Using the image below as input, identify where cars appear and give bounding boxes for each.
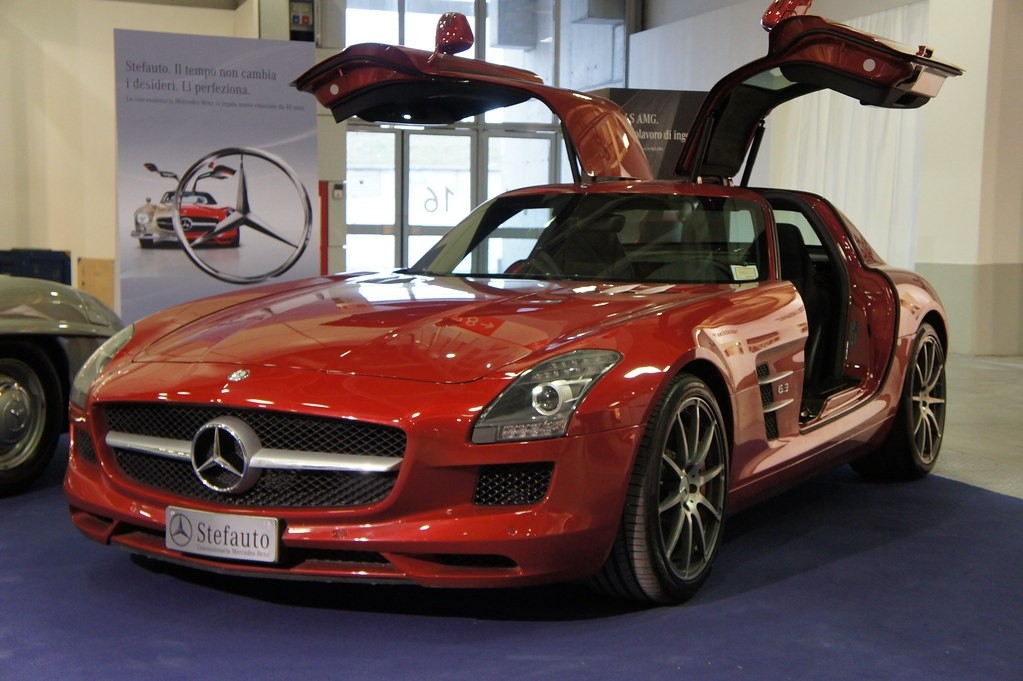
[0,272,130,499]
[129,162,240,249]
[72,0,949,614]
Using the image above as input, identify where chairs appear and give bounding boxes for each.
[535,200,635,280]
[738,222,820,360]
